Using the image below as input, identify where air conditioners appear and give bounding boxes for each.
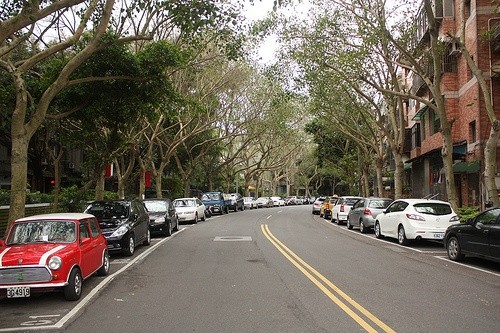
[449,42,459,56]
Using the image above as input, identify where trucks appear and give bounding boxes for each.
[203,192,232,217]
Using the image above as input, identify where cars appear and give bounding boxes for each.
[80,199,150,257]
[243,196,259,210]
[227,193,244,212]
[283,195,316,206]
[144,198,179,238]
[172,198,208,225]
[373,199,459,247]
[320,194,338,220]
[443,207,500,264]
[312,195,325,214]
[271,197,286,207]
[346,198,395,233]
[256,197,274,208]
[330,196,363,225]
[0,213,110,303]
